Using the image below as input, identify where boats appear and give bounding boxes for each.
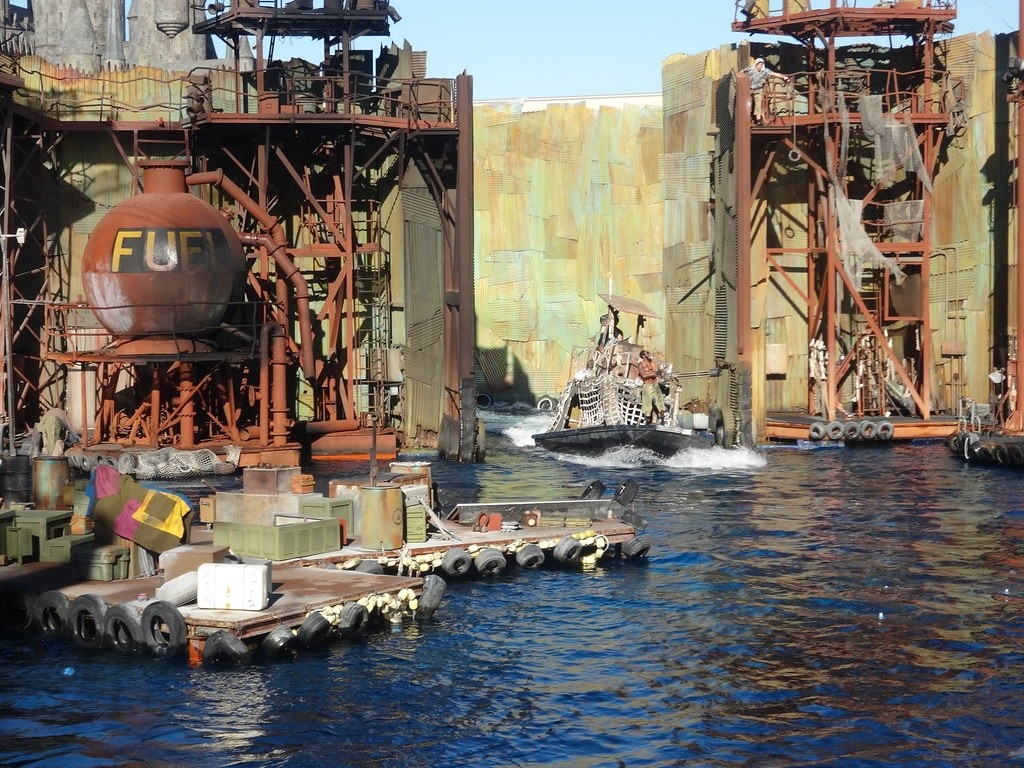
[533,298,720,467]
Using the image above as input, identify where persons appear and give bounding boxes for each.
[639,350,667,425]
[740,57,790,126]
